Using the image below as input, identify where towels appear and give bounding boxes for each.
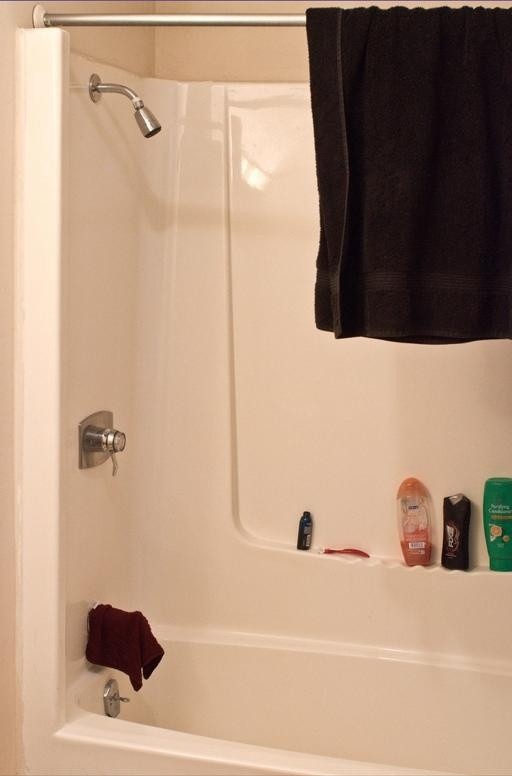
[86,603,164,692]
[301,8,511,342]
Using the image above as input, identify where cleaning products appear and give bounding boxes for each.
[297,512,312,549]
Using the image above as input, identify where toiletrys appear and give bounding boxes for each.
[296,510,313,551]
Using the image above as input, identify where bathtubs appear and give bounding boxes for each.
[56,623,508,773]
